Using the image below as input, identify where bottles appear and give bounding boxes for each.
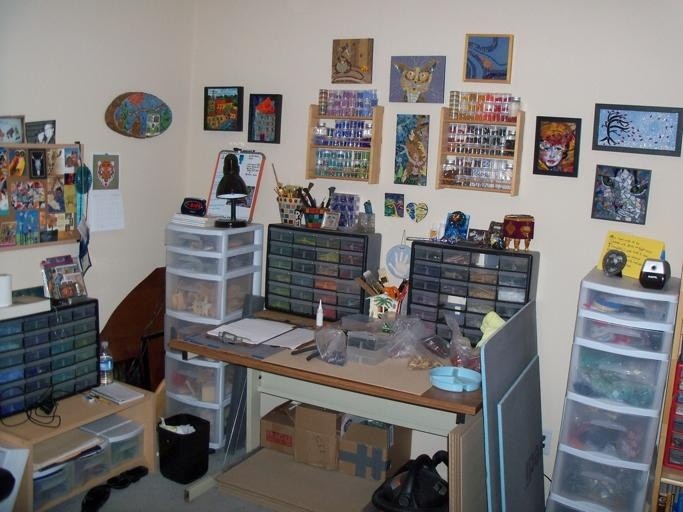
[447,124,514,155]
[314,120,372,148]
[100,340,113,384]
[443,156,513,190]
[314,149,369,179]
[449,90,520,123]
[318,88,377,117]
[316,299,323,327]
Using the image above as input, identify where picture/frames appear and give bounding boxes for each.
[591,103,683,157]
[0,143,86,250]
[462,32,515,85]
[532,115,581,178]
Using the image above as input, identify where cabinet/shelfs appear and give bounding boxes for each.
[434,106,525,197]
[0,380,157,512]
[540,267,683,511]
[162,211,264,457]
[305,104,384,186]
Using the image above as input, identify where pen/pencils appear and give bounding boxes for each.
[291,347,317,355]
[276,186,301,197]
[306,353,318,361]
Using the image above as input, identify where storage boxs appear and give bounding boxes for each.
[258,396,415,483]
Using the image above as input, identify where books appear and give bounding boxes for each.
[91,381,146,408]
[39,253,89,301]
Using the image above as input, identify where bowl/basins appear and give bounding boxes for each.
[428,366,481,392]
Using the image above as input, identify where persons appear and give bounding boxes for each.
[0,122,81,186]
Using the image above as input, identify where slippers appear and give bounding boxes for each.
[83,486,110,511]
[105,466,148,490]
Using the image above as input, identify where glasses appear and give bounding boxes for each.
[218,331,256,350]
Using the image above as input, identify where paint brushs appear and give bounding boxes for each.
[303,183,314,200]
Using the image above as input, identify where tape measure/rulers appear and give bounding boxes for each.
[355,277,376,296]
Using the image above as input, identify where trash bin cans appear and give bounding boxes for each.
[157,413,209,484]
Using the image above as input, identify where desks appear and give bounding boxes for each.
[168,310,484,512]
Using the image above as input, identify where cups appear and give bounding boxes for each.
[0,274,12,307]
